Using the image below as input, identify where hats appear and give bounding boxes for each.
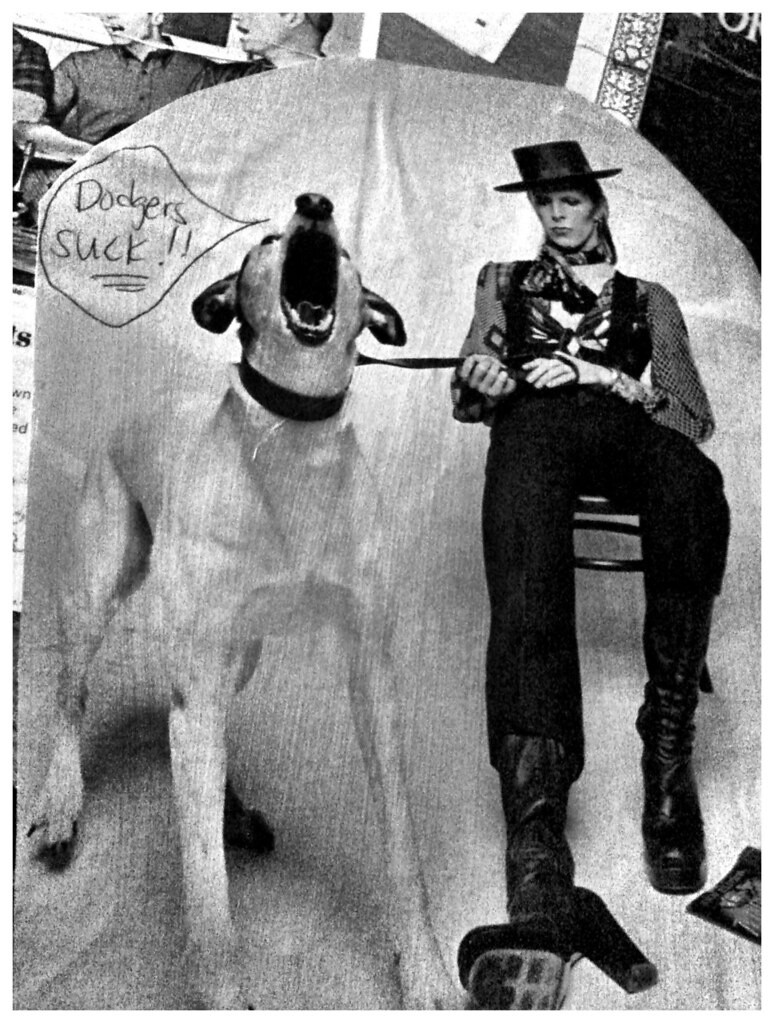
[493,139,622,193]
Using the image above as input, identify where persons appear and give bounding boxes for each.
[447,140,731,921]
[12,13,337,227]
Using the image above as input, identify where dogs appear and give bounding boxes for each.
[26,191,474,1010]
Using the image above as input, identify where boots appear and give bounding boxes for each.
[635,594,711,892]
[457,731,657,1009]
